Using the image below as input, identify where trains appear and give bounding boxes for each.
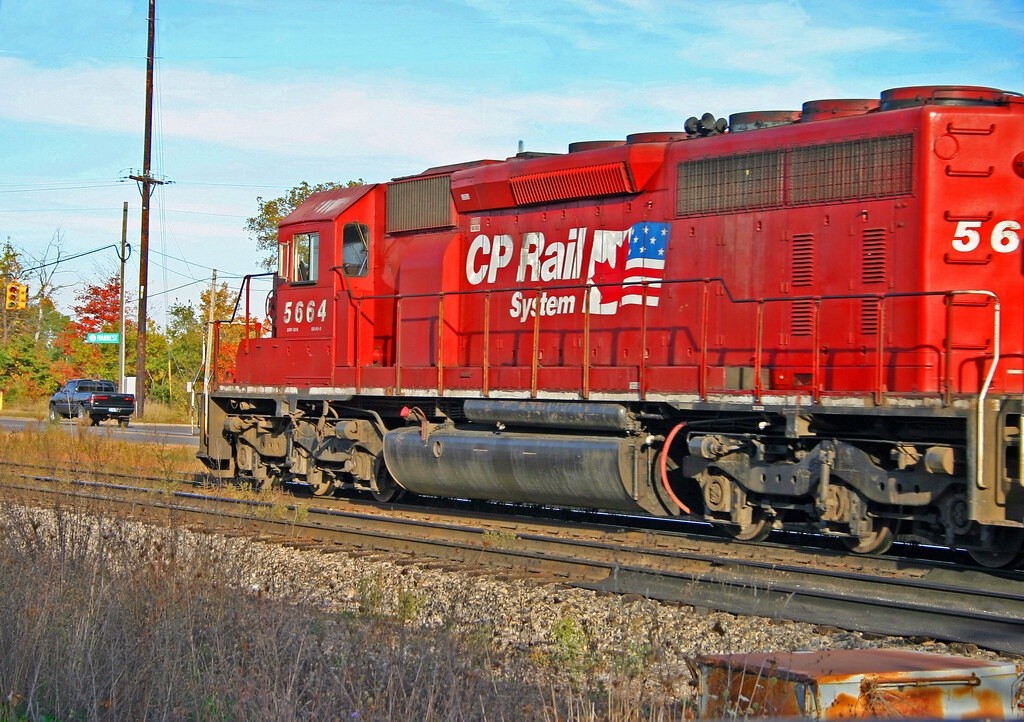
[199,88,1024,570]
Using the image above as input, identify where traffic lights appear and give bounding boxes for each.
[18,285,29,310]
[6,283,18,310]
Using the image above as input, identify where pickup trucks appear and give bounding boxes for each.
[49,382,135,430]
